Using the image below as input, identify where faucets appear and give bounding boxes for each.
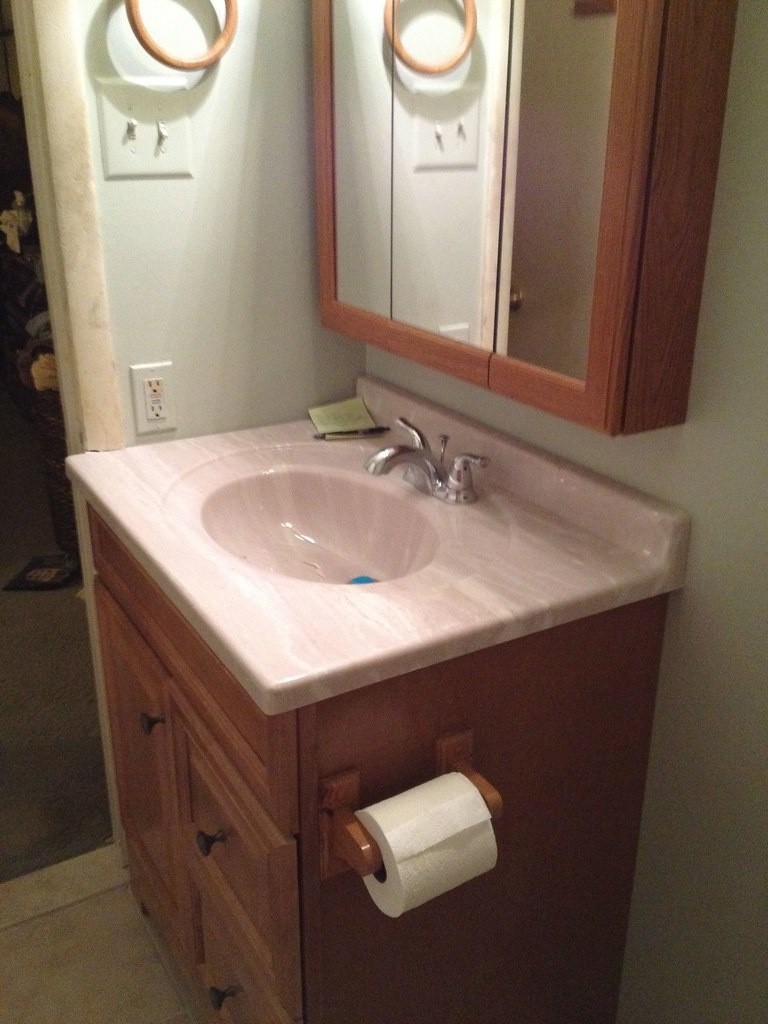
[361,416,492,505]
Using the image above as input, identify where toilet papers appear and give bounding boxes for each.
[353,771,499,919]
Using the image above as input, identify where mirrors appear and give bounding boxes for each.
[300,0,738,441]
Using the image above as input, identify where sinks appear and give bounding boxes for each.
[199,470,443,586]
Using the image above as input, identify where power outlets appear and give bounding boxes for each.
[129,356,180,440]
[438,321,472,347]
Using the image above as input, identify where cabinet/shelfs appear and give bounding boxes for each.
[80,499,676,1024]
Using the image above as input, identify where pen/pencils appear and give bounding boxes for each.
[314,426,390,439]
